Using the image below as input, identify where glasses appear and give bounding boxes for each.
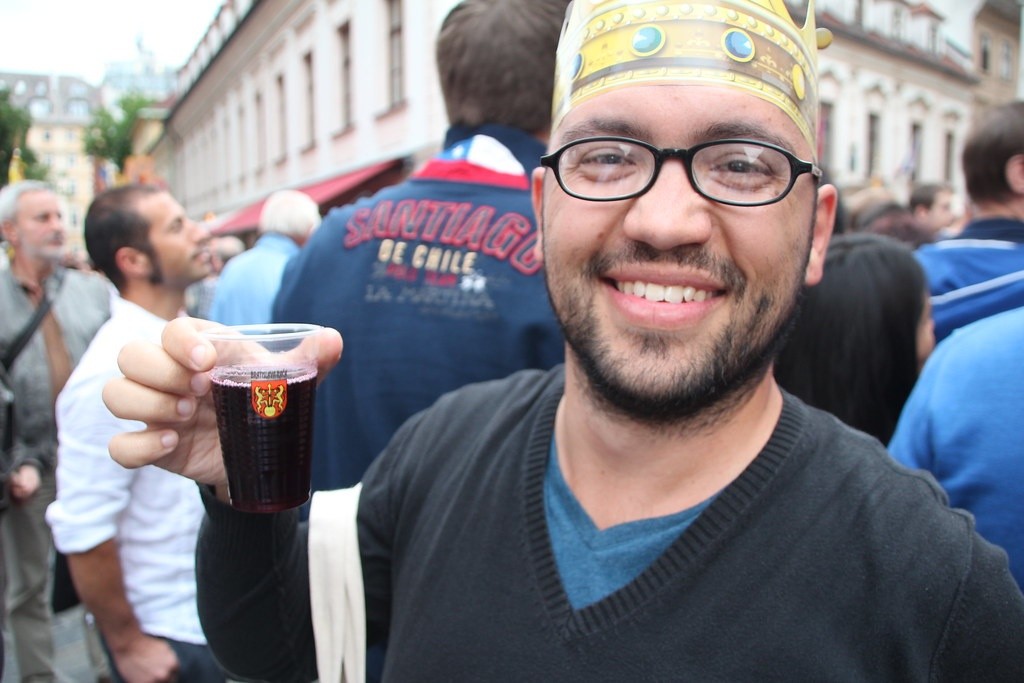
[542,137,824,208]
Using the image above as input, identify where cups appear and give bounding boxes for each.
[198,323,325,513]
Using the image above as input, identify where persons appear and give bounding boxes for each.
[0,0,1024,683]
